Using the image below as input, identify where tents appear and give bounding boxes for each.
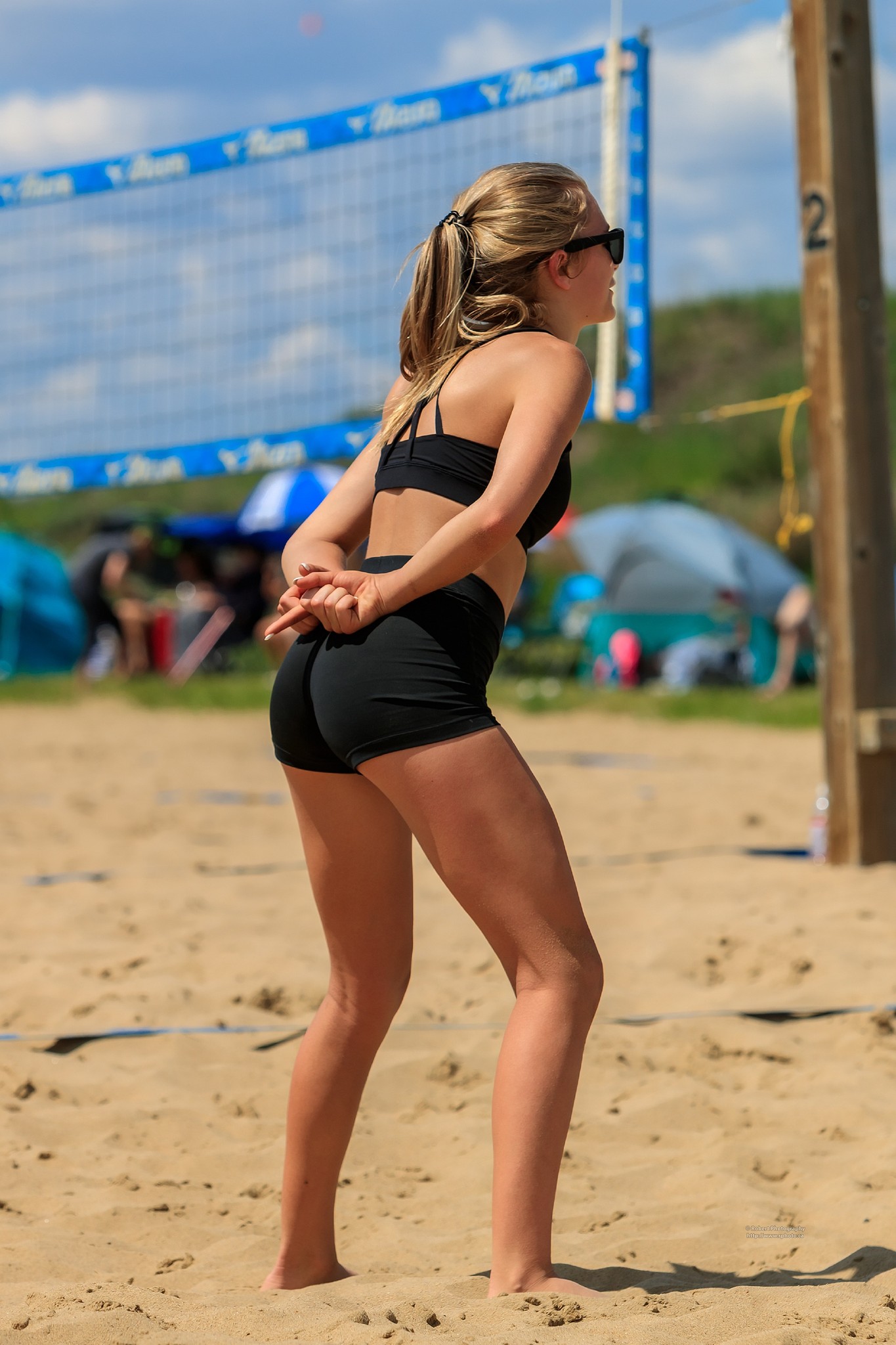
[562,501,820,685]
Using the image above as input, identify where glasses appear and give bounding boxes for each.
[520,226,625,277]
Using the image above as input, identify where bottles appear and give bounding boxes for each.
[811,786,829,860]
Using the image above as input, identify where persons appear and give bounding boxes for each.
[265,162,624,1298]
[72,526,301,685]
